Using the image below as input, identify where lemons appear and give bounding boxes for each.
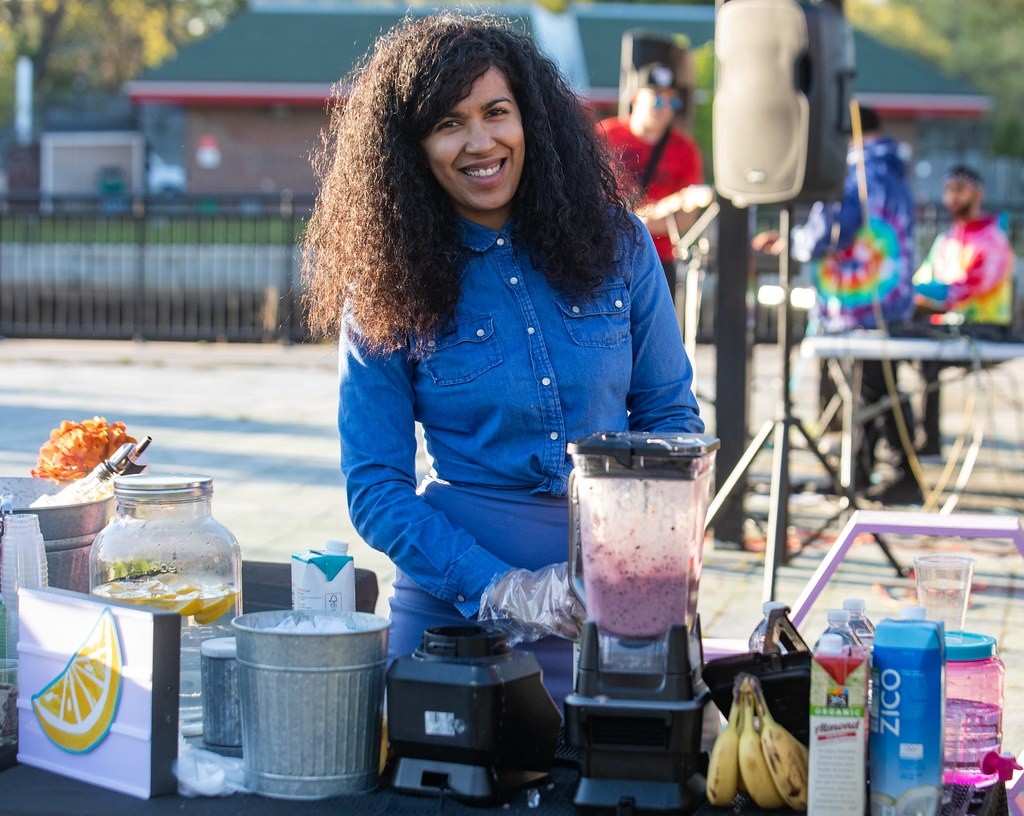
[107,579,239,624]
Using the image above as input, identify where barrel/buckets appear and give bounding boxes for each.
[234,612,391,799]
[0,476,114,591]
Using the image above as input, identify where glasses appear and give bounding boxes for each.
[650,94,683,112]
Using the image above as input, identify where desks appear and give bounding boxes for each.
[0,611,784,816]
[809,335,1024,518]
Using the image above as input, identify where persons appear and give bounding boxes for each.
[814,103,927,506]
[752,198,850,433]
[904,163,1016,457]
[585,59,702,310]
[305,8,709,717]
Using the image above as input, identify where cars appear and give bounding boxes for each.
[676,218,807,347]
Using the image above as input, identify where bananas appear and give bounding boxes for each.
[705,672,809,811]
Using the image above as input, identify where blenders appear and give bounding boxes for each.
[563,430,719,809]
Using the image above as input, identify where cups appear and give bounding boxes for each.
[913,554,974,632]
[0,514,49,772]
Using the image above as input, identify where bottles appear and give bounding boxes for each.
[808,611,869,816]
[870,609,1006,816]
[841,597,874,784]
[748,603,786,654]
[88,475,241,737]
